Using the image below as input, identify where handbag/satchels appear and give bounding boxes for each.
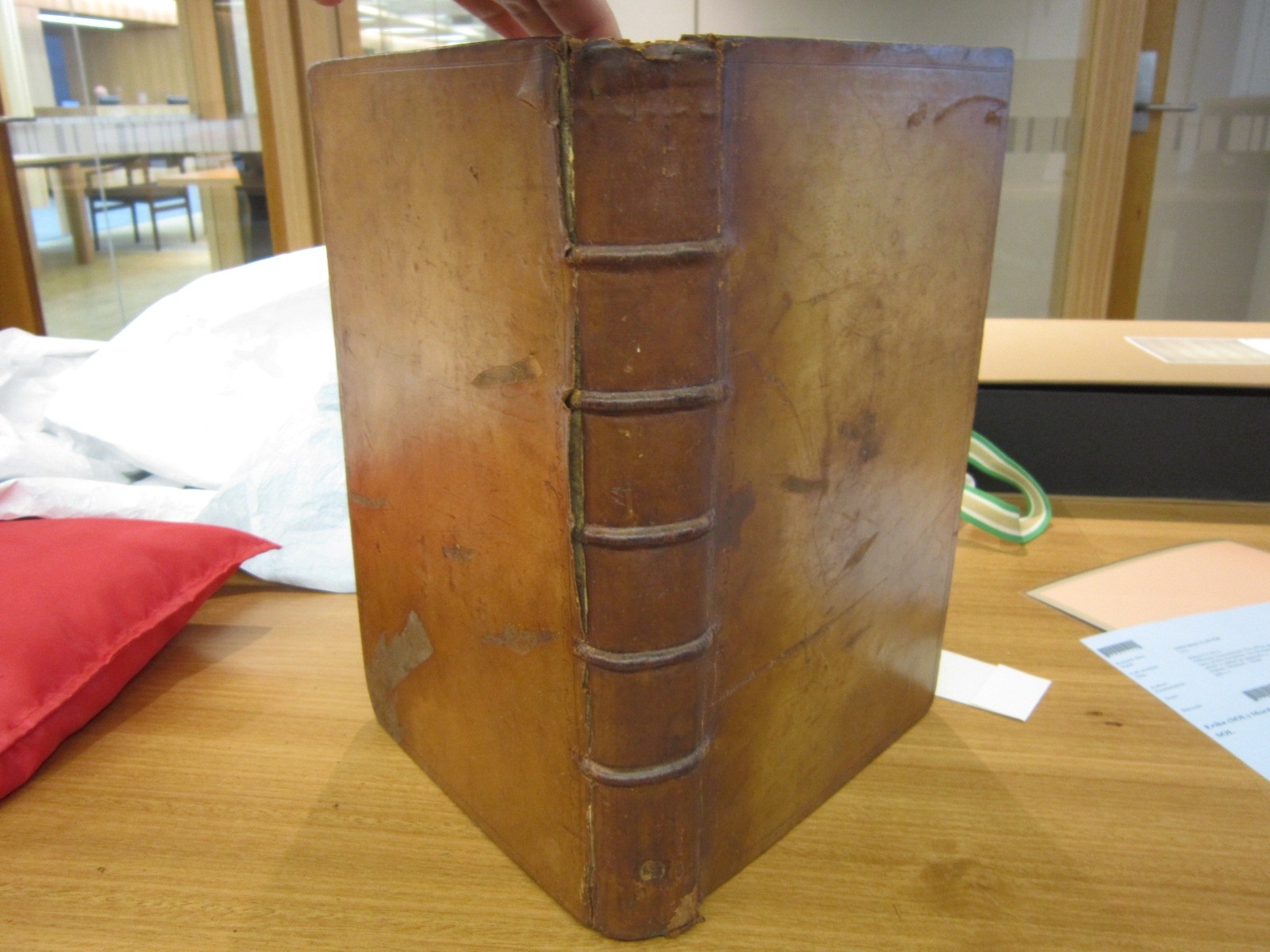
[36,242,1052,544]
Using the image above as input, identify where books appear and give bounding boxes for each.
[305,33,1015,941]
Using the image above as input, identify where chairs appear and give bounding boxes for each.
[86,155,196,249]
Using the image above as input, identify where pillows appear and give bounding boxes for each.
[1,518,281,800]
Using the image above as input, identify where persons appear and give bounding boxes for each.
[91,84,110,104]
